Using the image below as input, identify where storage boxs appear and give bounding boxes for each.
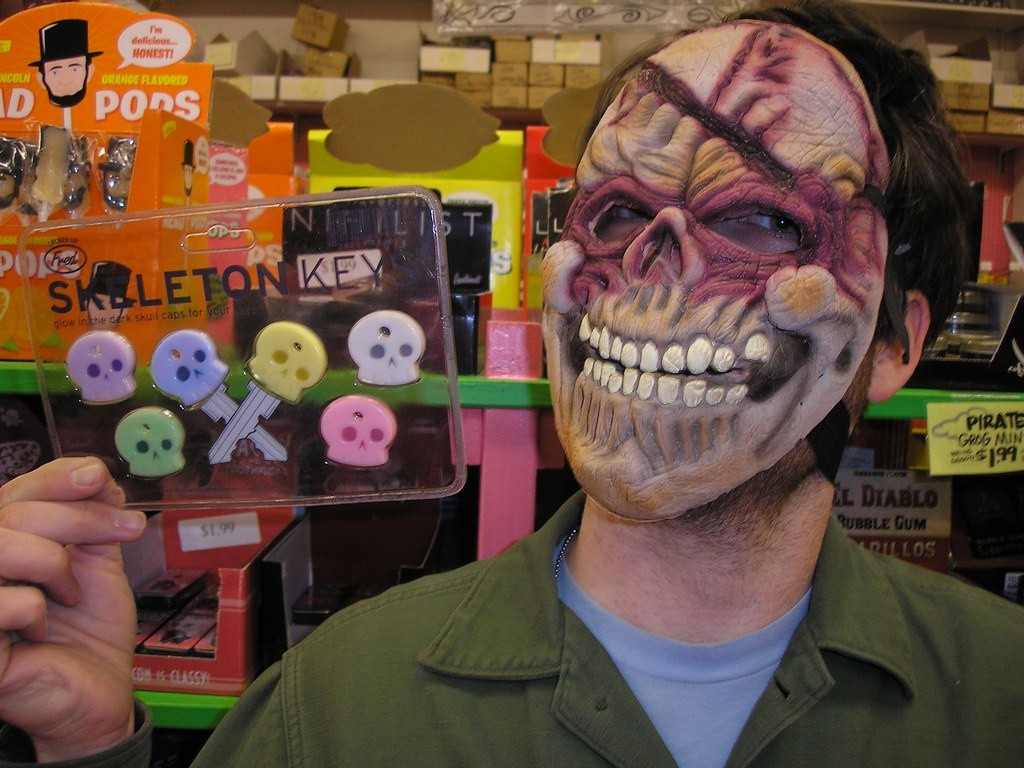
[200,3,618,375]
[120,502,313,699]
[909,26,1024,140]
[0,0,214,365]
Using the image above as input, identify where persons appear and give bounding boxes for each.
[1,0,1024,767]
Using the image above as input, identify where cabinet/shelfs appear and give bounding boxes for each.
[0,360,1024,733]
